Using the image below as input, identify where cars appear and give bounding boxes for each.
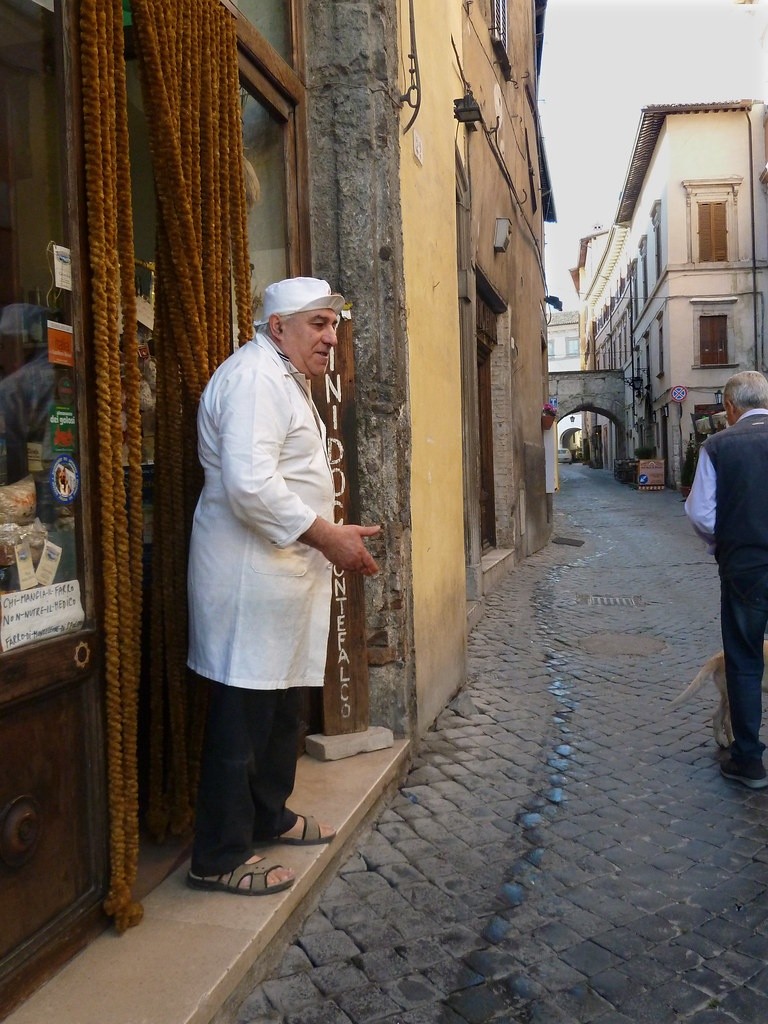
[558,448,572,464]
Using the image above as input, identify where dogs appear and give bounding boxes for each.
[666,638,768,747]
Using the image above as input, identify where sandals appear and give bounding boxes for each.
[257,813,337,844]
[186,854,294,893]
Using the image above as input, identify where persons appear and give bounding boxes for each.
[684,370,768,789]
[186,276,382,896]
[0,303,76,580]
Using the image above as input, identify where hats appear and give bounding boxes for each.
[254,277,345,327]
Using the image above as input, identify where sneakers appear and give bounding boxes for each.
[719,756,768,788]
